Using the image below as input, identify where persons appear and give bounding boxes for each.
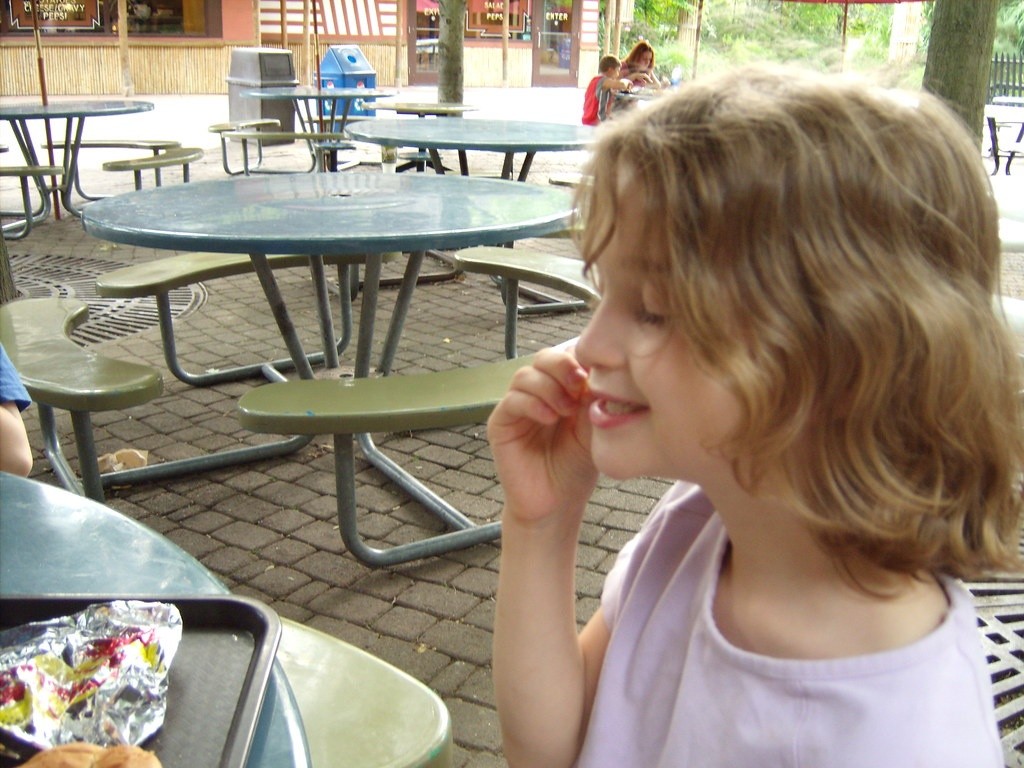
[484,66,1024,767]
[0,342,33,478]
[606,40,662,115]
[581,55,633,127]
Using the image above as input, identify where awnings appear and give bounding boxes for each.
[416,0,440,15]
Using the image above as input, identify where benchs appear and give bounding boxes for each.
[1,104,603,568]
[275,617,452,768]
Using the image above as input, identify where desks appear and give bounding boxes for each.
[0,101,154,198]
[252,88,394,169]
[345,118,601,274]
[0,469,311,768]
[80,171,585,376]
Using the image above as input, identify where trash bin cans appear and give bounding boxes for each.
[315,44,378,139]
[224,47,300,146]
